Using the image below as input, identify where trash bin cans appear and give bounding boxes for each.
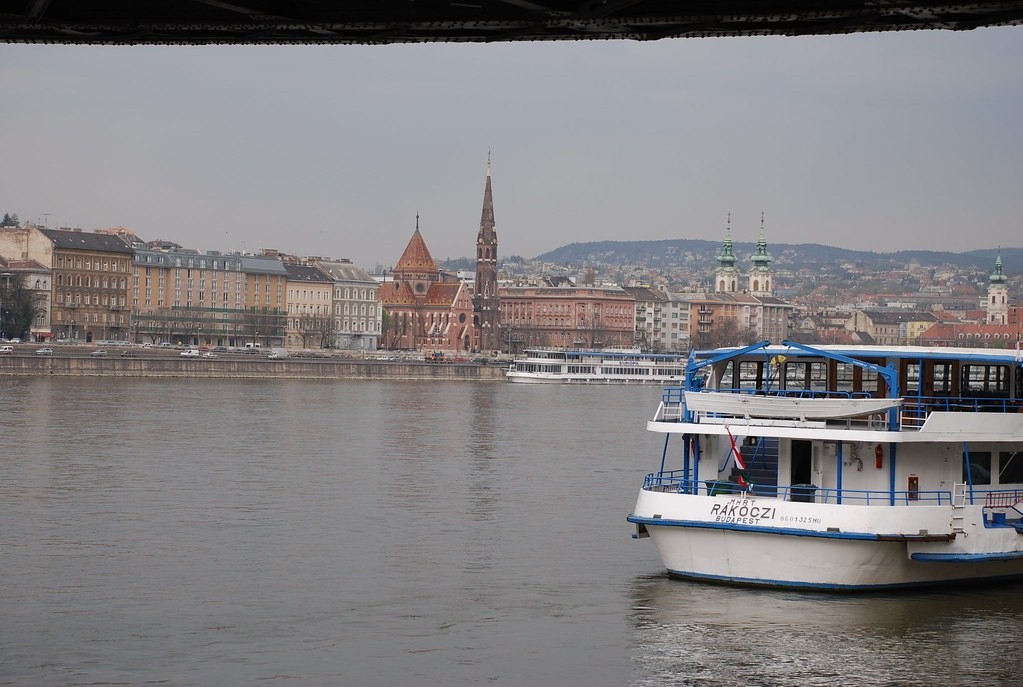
[790,484,818,503]
[705,480,733,496]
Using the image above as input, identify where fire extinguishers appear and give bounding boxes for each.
[875,443,882,468]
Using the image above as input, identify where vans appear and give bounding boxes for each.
[179,349,200,358]
[246,342,261,349]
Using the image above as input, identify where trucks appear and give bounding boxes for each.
[269,347,288,360]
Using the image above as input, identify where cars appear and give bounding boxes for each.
[162,342,171,348]
[119,341,132,347]
[105,340,116,346]
[364,355,425,361]
[198,347,209,352]
[202,352,218,360]
[144,343,153,346]
[176,344,191,350]
[90,349,107,357]
[211,346,226,353]
[0,337,22,344]
[36,348,53,356]
[122,350,137,358]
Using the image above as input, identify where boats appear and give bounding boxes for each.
[722,363,817,388]
[505,348,711,386]
[623,339,1023,596]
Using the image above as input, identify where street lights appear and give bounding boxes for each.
[226,324,230,355]
[198,328,201,347]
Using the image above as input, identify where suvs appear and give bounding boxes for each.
[0,346,14,355]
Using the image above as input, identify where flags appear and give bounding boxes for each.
[729,432,754,491]
